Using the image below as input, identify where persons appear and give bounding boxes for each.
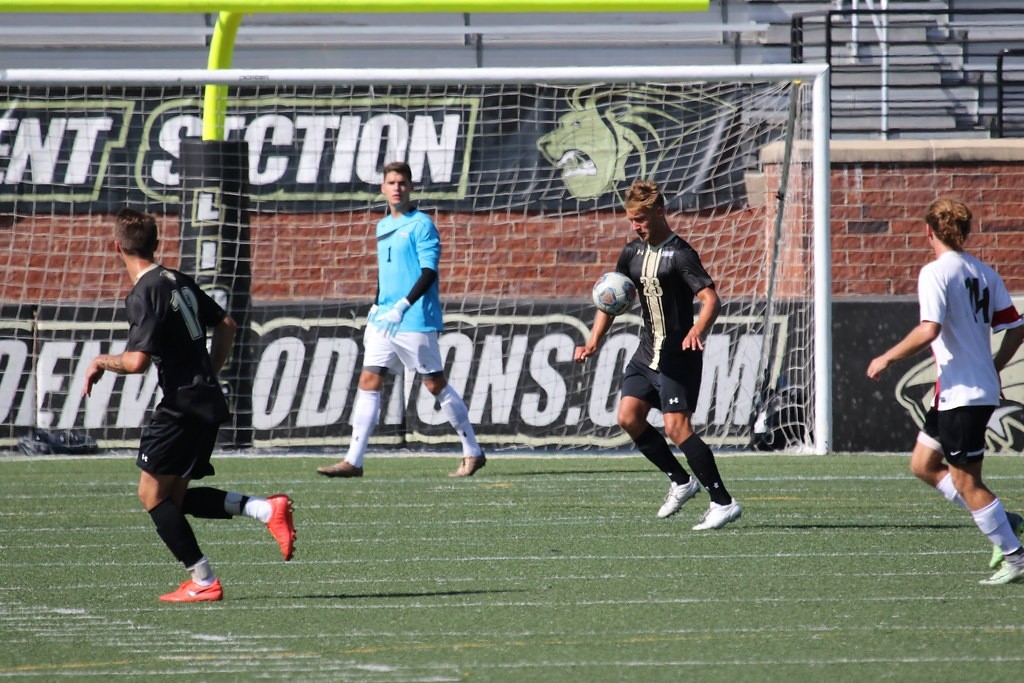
[574,182,741,531]
[317,163,486,479]
[868,197,1023,587]
[83,211,297,602]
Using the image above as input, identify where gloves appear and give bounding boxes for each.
[367,303,379,321]
[376,296,412,338]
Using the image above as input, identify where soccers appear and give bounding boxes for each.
[592,271,636,316]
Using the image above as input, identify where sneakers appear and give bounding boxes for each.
[160,577,223,602]
[691,496,742,530]
[656,475,701,519]
[977,511,1024,586]
[447,449,487,477]
[317,460,364,478]
[265,494,298,562]
